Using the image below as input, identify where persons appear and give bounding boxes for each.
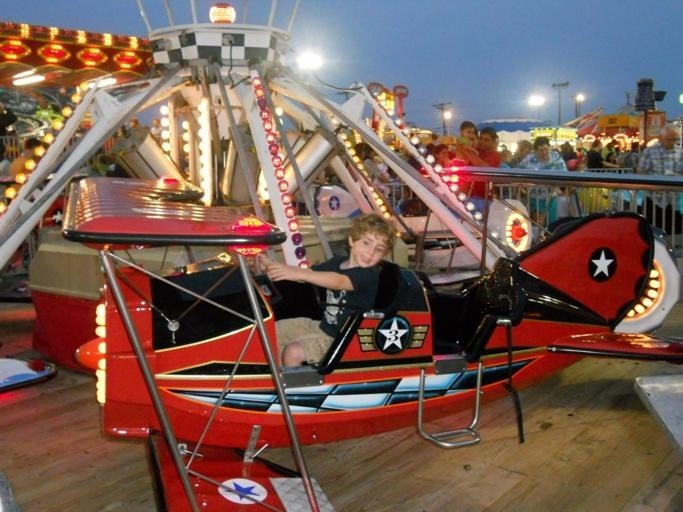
[8,136,40,202]
[265,212,399,371]
[0,142,12,197]
